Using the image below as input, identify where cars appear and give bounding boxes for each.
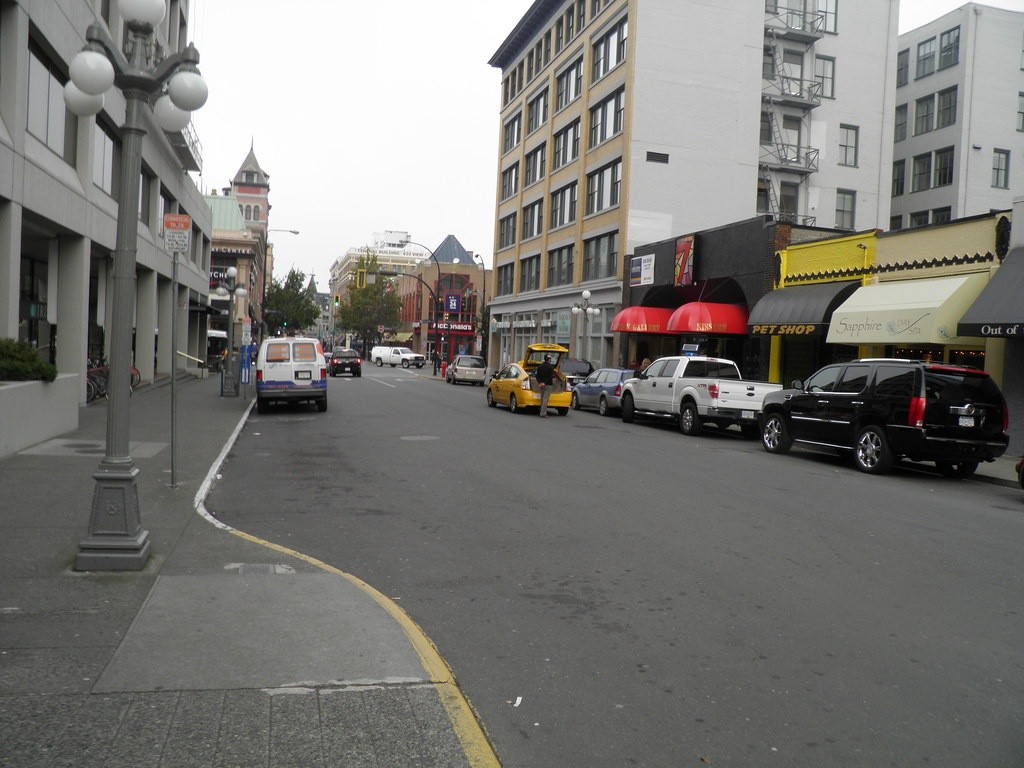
[486,343,572,416]
[539,358,595,392]
[445,356,486,387]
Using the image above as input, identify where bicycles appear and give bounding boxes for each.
[86,356,140,403]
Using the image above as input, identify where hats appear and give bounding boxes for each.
[544,354,552,358]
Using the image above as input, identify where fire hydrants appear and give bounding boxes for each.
[440,362,447,378]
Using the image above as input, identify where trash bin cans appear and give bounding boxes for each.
[225,352,242,395]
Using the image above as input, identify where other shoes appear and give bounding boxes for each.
[540,415,549,418]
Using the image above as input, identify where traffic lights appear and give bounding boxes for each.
[334,295,340,306]
[282,320,287,326]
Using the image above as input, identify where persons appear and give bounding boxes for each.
[430,347,441,375]
[639,357,651,370]
[535,354,554,418]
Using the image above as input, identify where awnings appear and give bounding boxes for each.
[609,246,1024,359]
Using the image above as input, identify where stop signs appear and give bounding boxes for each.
[378,326,384,332]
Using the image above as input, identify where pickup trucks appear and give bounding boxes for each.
[371,346,426,369]
[328,349,362,377]
[620,356,783,440]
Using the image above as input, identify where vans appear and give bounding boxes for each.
[205,330,227,372]
[257,338,328,414]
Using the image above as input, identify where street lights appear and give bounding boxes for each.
[259,229,300,344]
[475,254,485,358]
[400,240,441,377]
[571,289,600,359]
[61,1,210,570]
[216,267,248,397]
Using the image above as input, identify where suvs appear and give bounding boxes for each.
[572,366,638,416]
[756,359,1010,479]
